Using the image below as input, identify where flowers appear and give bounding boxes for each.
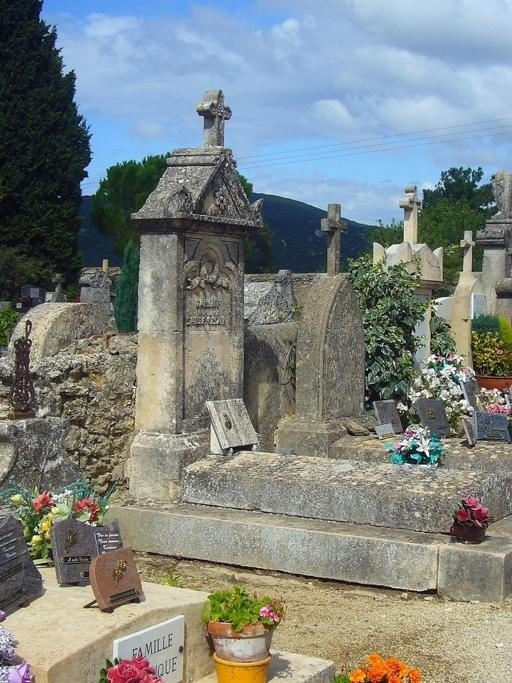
[202,584,281,633]
[337,653,420,682]
[383,424,446,465]
[0,609,35,683]
[453,495,490,530]
[10,483,119,568]
[99,655,163,682]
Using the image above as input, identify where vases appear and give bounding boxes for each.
[212,653,275,683]
[204,621,273,664]
[452,528,484,543]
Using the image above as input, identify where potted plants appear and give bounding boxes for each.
[472,330,512,391]
